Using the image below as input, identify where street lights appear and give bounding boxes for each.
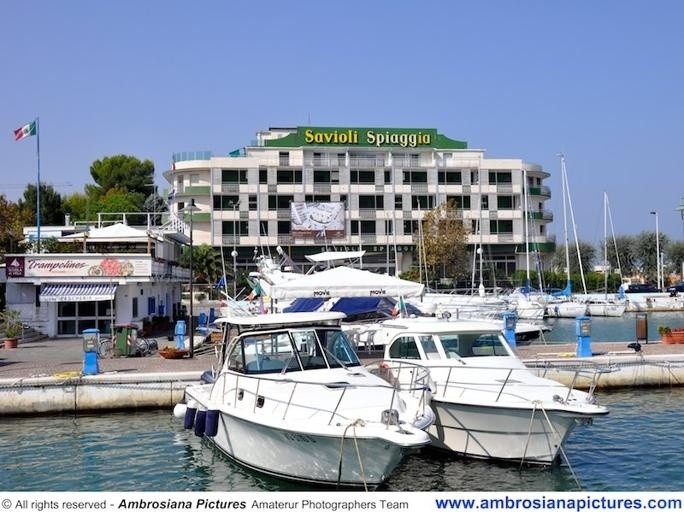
[182,198,204,353]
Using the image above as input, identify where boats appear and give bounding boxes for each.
[171,311,442,493]
[354,308,616,475]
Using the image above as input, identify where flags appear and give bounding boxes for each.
[13,120,36,143]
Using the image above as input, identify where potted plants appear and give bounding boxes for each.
[671,326,683,344]
[656,325,674,344]
[1,305,24,350]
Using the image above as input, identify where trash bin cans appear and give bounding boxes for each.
[635,313,649,344]
[114,322,139,358]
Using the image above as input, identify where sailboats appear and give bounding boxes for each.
[221,154,684,343]
